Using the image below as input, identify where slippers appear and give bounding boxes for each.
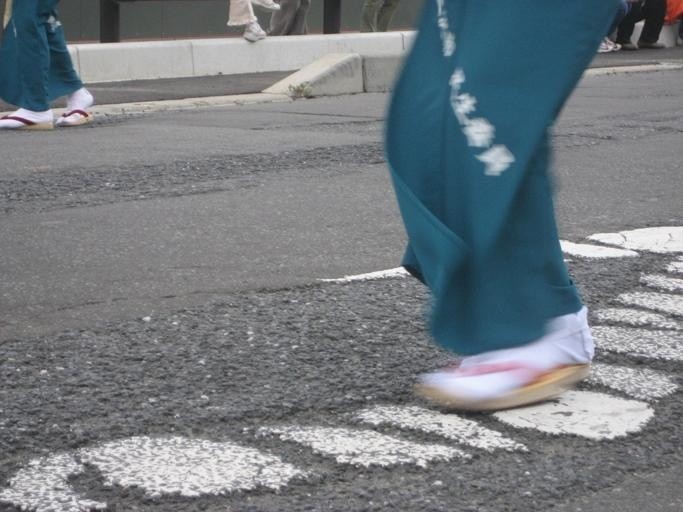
[415,363,591,411]
[1,110,92,129]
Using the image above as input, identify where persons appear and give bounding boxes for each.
[227,0,281,41]
[383,0,632,412]
[595,0,682,54]
[0,0,94,131]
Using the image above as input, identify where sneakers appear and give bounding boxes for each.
[676,34,682,44]
[243,1,281,41]
[597,37,665,53]
